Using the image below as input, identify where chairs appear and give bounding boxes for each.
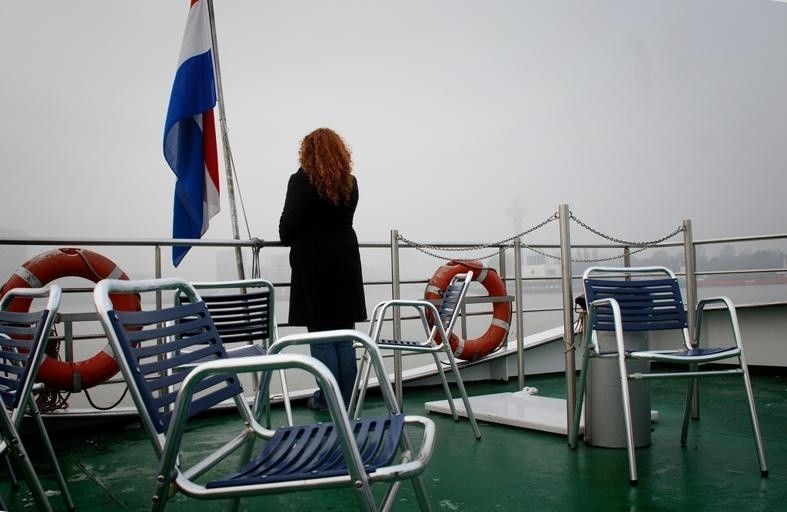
[0,281,76,512]
[164,277,296,434]
[346,267,484,444]
[91,274,439,511]
[566,264,772,488]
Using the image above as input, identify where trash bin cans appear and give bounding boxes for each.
[576,295,651,448]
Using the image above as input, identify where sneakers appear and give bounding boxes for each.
[307,395,350,410]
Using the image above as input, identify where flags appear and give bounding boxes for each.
[163,0,221,268]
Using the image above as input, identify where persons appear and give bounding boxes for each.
[280,128,367,412]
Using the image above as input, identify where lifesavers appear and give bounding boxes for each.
[425,259,510,361]
[0,248,143,392]
